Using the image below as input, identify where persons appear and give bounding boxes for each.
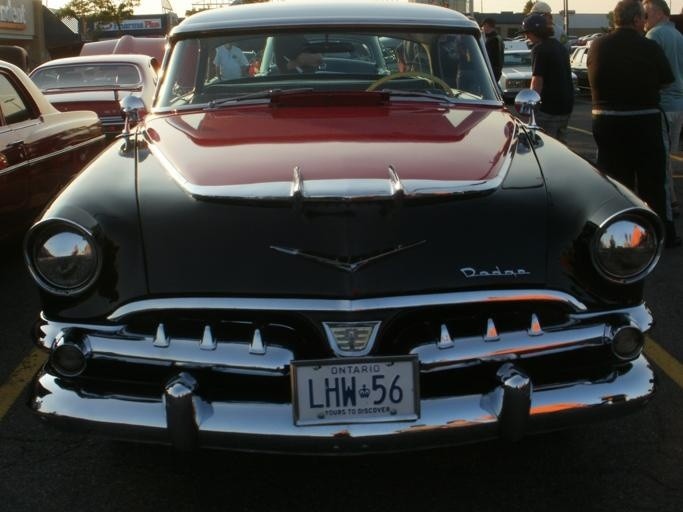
[397,0,505,82]
[528,1,569,51]
[642,0,682,235]
[213,40,249,80]
[514,13,575,145]
[271,34,324,75]
[587,0,675,218]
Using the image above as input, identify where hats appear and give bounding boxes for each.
[519,1,554,36]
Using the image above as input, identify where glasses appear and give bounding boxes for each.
[645,12,649,20]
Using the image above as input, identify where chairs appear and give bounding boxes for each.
[55,69,137,84]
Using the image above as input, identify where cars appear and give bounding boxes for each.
[26,35,197,141]
[1,60,106,242]
[19,0,666,464]
[565,33,604,93]
[497,41,580,99]
